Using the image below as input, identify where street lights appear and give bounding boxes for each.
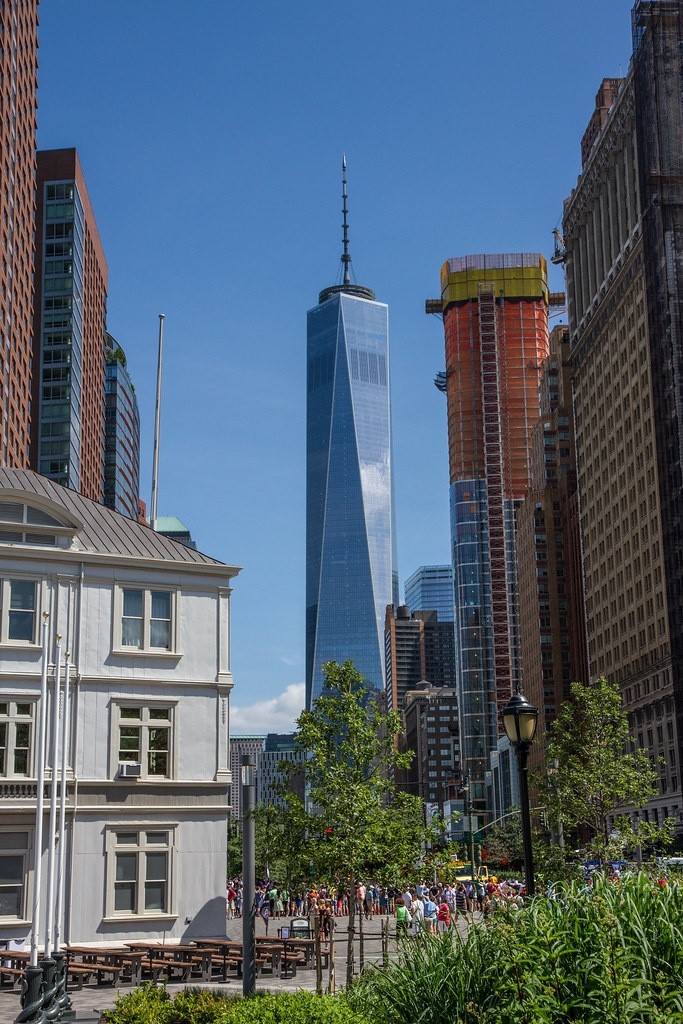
[460,777,478,880]
[500,695,539,898]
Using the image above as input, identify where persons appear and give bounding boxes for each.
[578,877,594,896]
[227,873,555,951]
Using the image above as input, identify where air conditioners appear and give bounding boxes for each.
[118,762,142,779]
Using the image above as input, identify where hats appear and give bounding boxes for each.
[358,882,362,885]
[319,898,325,904]
[478,882,483,886]
[438,883,442,886]
[446,884,449,886]
[369,885,374,888]
[311,898,317,902]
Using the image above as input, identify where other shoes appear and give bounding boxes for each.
[272,911,394,921]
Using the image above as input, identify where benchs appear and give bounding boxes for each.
[0,935,340,993]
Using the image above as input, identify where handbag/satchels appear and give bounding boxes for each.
[405,908,412,922]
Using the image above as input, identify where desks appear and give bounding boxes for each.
[192,939,284,979]
[253,937,317,971]
[123,942,219,981]
[0,950,44,971]
[62,945,147,986]
